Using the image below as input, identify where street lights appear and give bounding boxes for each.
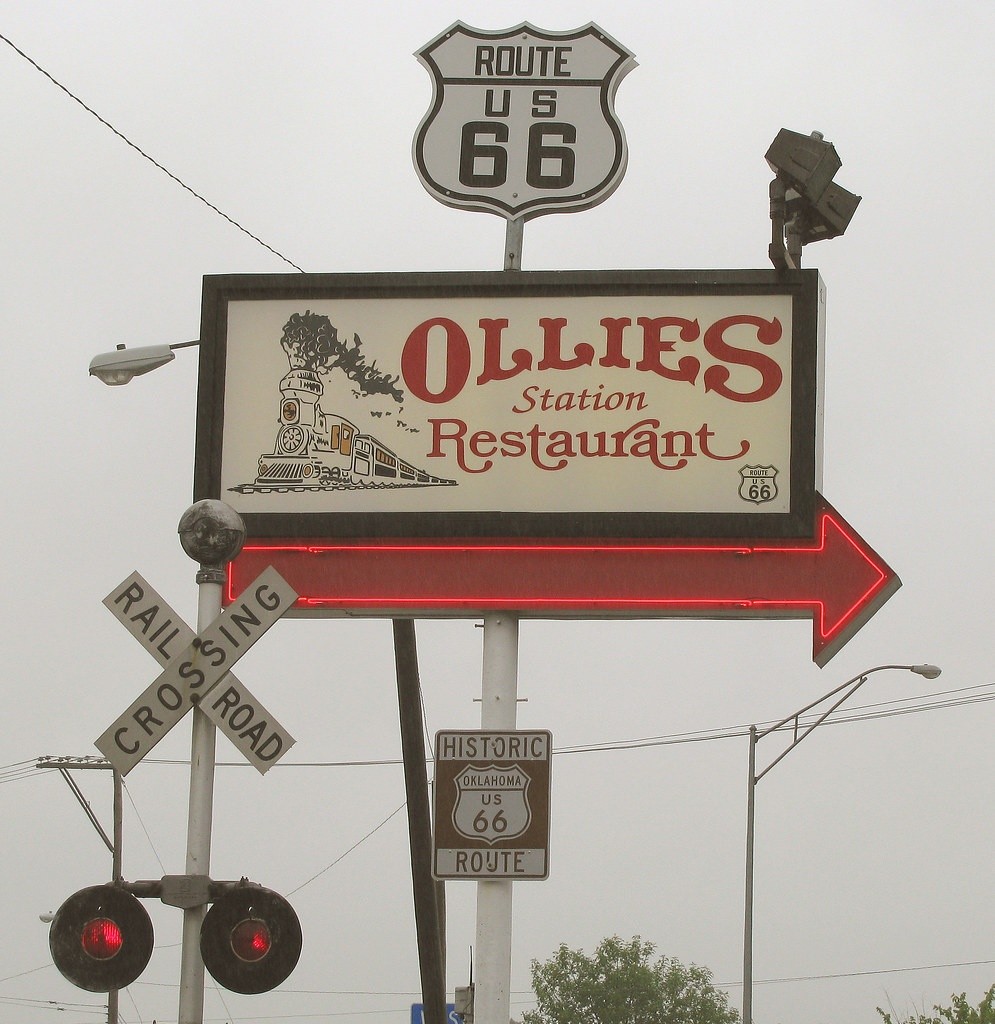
[742,664,943,1024]
[84,338,445,1024]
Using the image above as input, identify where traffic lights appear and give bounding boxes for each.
[49,884,154,994]
[197,887,302,995]
